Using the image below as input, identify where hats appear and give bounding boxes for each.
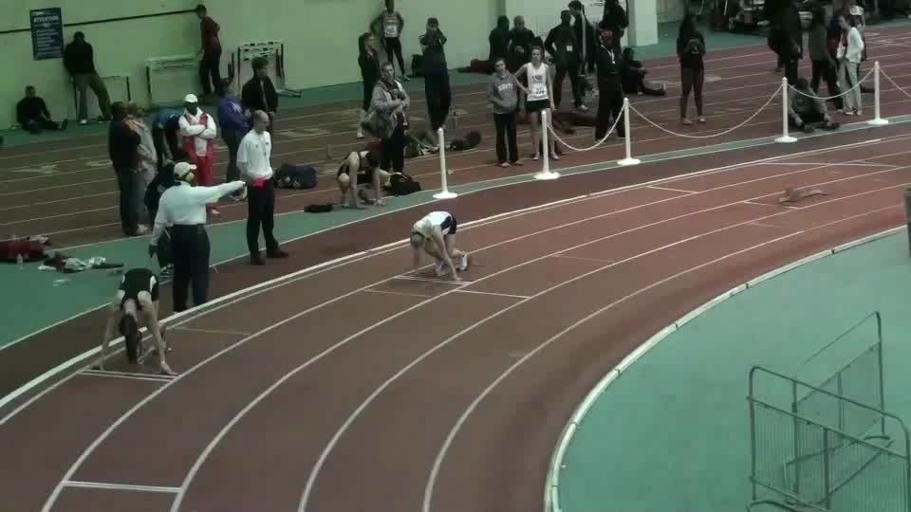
[173,160,199,181]
[213,75,234,94]
[184,92,200,104]
[601,30,619,41]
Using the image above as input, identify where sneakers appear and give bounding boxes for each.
[266,248,288,258]
[356,126,365,138]
[577,104,589,111]
[60,115,112,129]
[134,223,149,236]
[341,194,369,207]
[801,121,840,133]
[680,116,707,124]
[138,323,168,357]
[836,108,863,116]
[533,152,560,160]
[591,134,635,145]
[250,254,266,265]
[497,159,523,168]
[434,251,470,277]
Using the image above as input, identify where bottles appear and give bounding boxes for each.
[17,254,24,269]
[51,276,72,290]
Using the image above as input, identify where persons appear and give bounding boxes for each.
[676,15,707,123]
[487,1,666,167]
[335,1,454,211]
[763,0,868,134]
[1,2,289,375]
[409,210,468,280]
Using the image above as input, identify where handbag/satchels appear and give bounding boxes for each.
[450,130,481,150]
[272,162,318,189]
[362,109,397,139]
[0,238,42,262]
[384,172,422,197]
[410,53,425,78]
